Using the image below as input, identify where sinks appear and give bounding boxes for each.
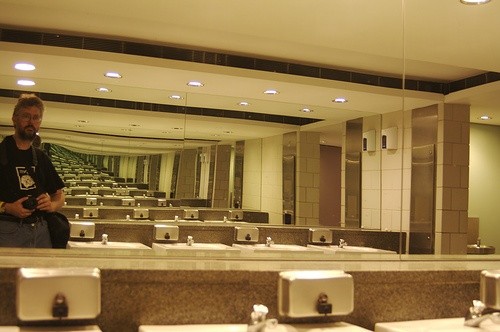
[140,318,372,331]
[49,143,251,225]
[67,240,152,250]
[374,314,499,332]
[231,243,325,259]
[308,245,398,254]
[153,241,241,258]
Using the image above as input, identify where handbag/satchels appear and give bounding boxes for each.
[47,211,70,249]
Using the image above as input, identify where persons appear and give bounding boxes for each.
[0,94,72,250]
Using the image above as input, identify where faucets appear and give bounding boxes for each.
[101,234,110,244]
[52,156,230,223]
[265,237,275,248]
[464,300,500,327]
[186,235,195,246]
[336,238,349,249]
[247,304,278,331]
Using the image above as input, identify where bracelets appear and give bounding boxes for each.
[0,201,6,214]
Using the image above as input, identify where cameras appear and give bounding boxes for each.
[22,195,38,210]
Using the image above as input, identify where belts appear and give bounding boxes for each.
[0,215,44,223]
